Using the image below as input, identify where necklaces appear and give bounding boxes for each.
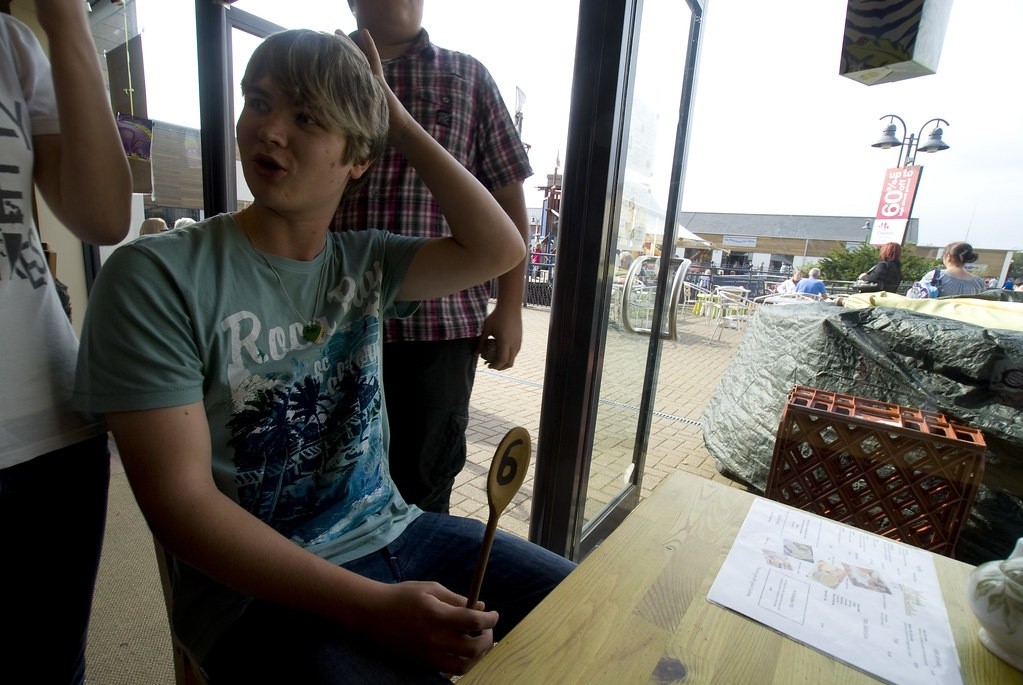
[240,207,330,344]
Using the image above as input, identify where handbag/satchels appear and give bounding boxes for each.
[910,267,941,298]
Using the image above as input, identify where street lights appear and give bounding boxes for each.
[871,114,953,168]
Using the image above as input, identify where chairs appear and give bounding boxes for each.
[607,272,851,348]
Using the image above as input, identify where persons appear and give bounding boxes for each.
[776,269,805,294]
[922,241,987,299]
[529,240,794,301]
[175,217,197,230]
[0,0,133,685]
[68,26,577,685]
[794,268,828,300]
[983,276,1023,293]
[140,219,169,239]
[857,241,902,294]
[327,1,533,513]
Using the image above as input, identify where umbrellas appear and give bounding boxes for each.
[616,203,711,255]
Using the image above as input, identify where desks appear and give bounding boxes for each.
[453,470,1023,685]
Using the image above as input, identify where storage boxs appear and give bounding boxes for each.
[764,385,987,563]
